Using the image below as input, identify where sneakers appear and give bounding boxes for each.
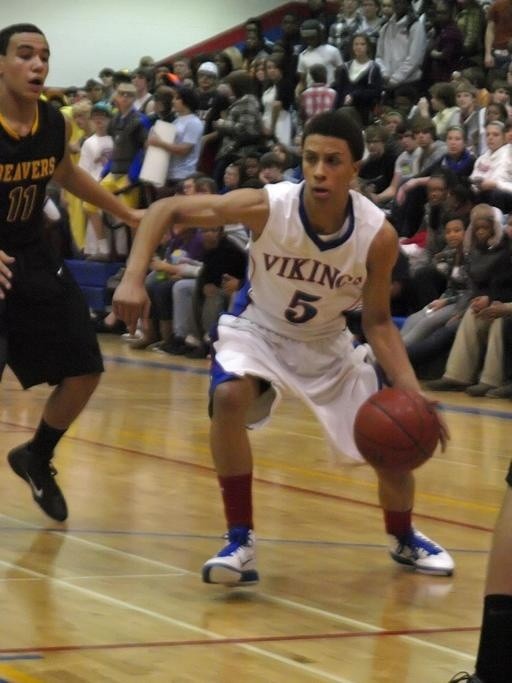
[424,377,467,391]
[203,527,261,586]
[183,343,208,360]
[467,384,496,397]
[165,341,191,355]
[7,443,68,520]
[486,380,512,399]
[388,526,455,575]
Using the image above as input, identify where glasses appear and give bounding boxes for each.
[67,93,78,97]
[132,75,146,79]
[118,90,137,98]
[198,73,216,79]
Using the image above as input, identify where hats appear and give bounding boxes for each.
[276,80,297,102]
[197,62,218,80]
[64,86,80,94]
[300,20,325,39]
[154,86,176,100]
[82,77,106,90]
[89,102,114,118]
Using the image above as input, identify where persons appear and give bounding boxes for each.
[112,115,455,585]
[134,88,171,205]
[427,3,459,78]
[480,106,507,148]
[243,17,275,56]
[377,0,424,94]
[329,0,361,48]
[0,22,168,522]
[428,125,472,190]
[347,1,384,58]
[47,51,170,103]
[477,0,498,68]
[373,121,421,210]
[299,64,336,122]
[134,146,298,361]
[237,31,268,70]
[424,202,512,333]
[84,85,147,255]
[67,101,92,157]
[391,117,448,215]
[396,212,465,351]
[183,60,228,177]
[146,85,204,243]
[455,83,480,143]
[421,293,512,400]
[265,11,301,61]
[469,119,509,189]
[413,174,458,240]
[444,36,511,99]
[479,83,512,128]
[334,35,379,119]
[354,124,392,199]
[213,70,262,181]
[293,19,343,99]
[475,448,512,680]
[411,82,461,137]
[379,0,397,24]
[453,2,484,80]
[260,56,291,139]
[161,41,253,97]
[79,103,123,257]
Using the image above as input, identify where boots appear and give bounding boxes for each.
[130,318,158,350]
[147,320,174,350]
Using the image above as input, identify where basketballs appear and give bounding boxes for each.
[352,386,441,479]
